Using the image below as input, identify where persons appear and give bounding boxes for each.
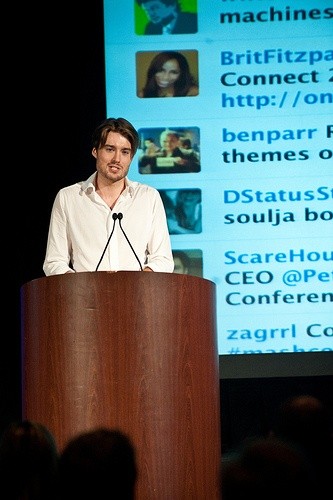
[43,117,174,277]
[137,130,201,174]
[172,250,190,274]
[166,189,201,234]
[136,50,198,97]
[135,0,198,35]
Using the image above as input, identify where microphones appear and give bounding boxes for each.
[96,213,118,271]
[118,213,143,271]
